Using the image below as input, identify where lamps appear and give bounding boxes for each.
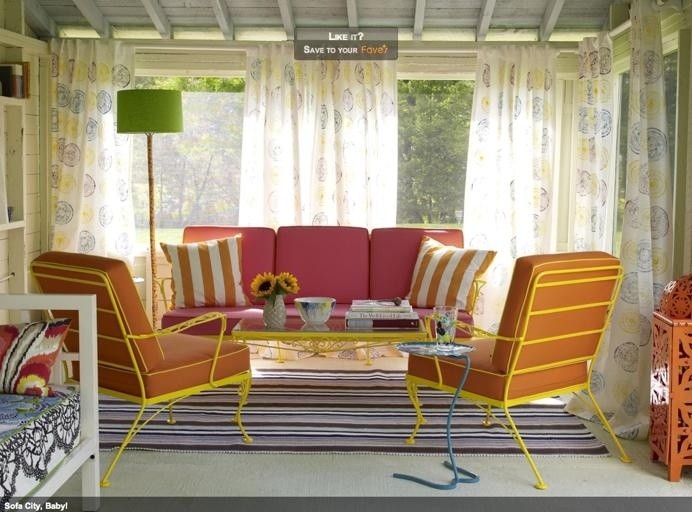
[116,88,185,328]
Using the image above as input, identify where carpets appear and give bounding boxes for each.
[99,366,607,455]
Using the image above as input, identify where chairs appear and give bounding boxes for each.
[409,250,636,488]
[30,249,254,488]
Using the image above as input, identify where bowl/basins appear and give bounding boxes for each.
[294,295,337,325]
[300,323,347,332]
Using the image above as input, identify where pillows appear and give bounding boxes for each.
[405,234,497,313]
[1,316,70,396]
[159,232,252,311]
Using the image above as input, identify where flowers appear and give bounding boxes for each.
[251,268,299,301]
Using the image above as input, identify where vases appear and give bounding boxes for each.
[261,295,287,329]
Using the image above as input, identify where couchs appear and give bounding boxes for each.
[161,224,476,343]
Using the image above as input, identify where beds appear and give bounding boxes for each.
[0,292,101,512]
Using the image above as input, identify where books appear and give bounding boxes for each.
[345,305,413,319]
[350,298,414,313]
[344,311,420,329]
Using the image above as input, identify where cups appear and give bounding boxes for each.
[433,306,459,351]
